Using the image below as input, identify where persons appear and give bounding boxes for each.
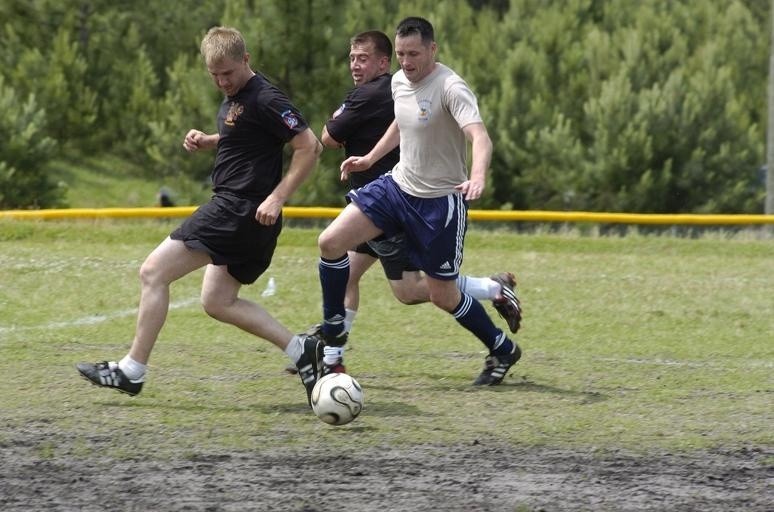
[74,26,326,409]
[296,16,522,387]
[320,30,523,346]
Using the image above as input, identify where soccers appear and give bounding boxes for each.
[311,373,364,425]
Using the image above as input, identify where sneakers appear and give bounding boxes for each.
[472,344,522,387]
[490,273,522,334]
[75,360,145,397]
[295,338,323,408]
[298,322,348,375]
